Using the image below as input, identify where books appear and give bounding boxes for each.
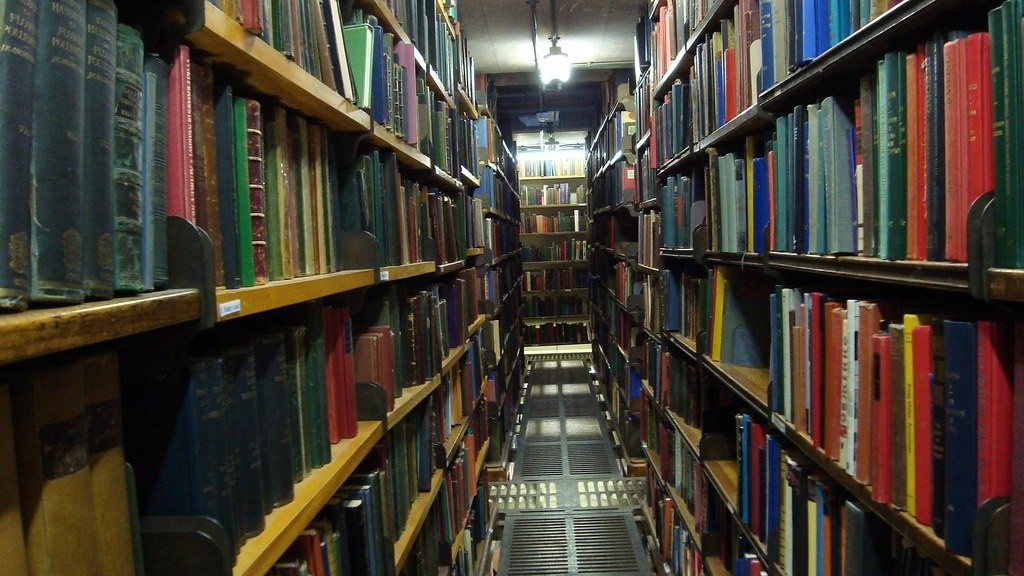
[0,1,1024,576]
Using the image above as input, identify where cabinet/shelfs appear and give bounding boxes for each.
[0,0,529,575]
[638,0,1023,576]
[589,95,647,477]
[517,143,592,347]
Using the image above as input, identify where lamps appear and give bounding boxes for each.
[537,1,571,85]
